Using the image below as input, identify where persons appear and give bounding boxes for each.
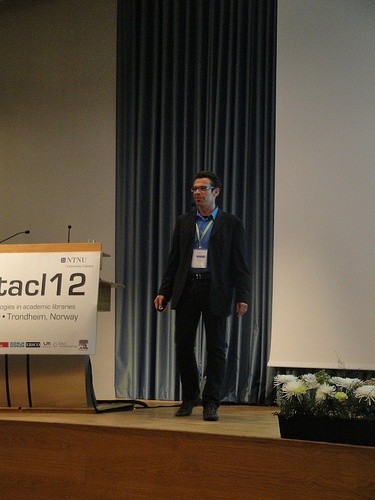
[154,171,247,420]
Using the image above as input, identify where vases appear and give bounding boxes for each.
[278,415,375,447]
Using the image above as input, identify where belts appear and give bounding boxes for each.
[187,272,213,281]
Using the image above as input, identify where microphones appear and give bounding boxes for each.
[68,225,71,243]
[0,230,30,243]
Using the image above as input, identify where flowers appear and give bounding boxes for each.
[274,370,375,421]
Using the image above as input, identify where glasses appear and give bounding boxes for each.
[191,185,215,192]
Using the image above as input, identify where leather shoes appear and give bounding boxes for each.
[202,404,219,421]
[174,390,202,417]
